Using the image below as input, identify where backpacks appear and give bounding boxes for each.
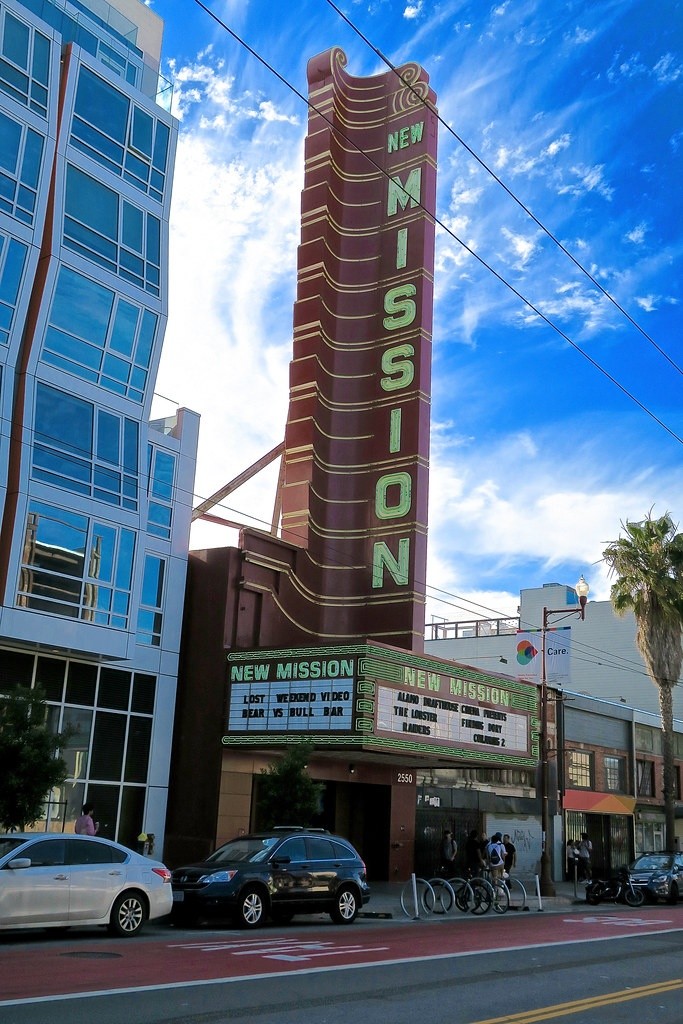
[491,844,501,865]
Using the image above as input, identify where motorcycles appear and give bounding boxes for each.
[585,864,645,906]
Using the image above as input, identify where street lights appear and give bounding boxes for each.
[537,573,589,904]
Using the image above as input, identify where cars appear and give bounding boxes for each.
[0,831,175,939]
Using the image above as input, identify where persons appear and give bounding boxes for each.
[75,803,98,835]
[465,830,517,901]
[440,830,457,884]
[568,833,592,884]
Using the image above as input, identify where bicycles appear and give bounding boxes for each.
[424,859,510,915]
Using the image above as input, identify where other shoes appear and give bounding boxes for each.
[588,880,592,884]
[581,879,589,883]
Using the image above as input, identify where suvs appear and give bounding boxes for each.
[625,850,683,904]
[169,824,372,929]
